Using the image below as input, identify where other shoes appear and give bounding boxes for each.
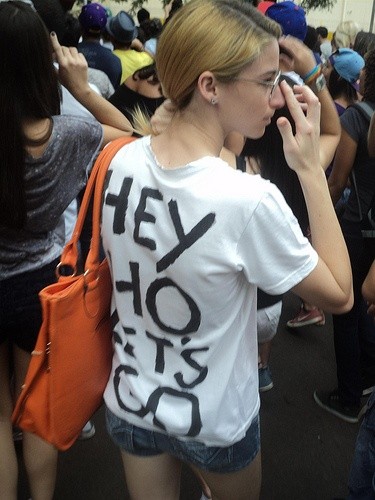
[314,390,362,422]
[258,365,274,392]
[289,308,325,327]
[80,423,96,439]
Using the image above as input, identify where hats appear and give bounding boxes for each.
[333,20,360,47]
[106,10,138,43]
[329,47,365,91]
[264,1,307,40]
[78,3,108,29]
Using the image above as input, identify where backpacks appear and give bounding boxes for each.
[346,101,375,162]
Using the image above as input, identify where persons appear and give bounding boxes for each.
[0,1,375,500]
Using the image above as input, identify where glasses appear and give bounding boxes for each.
[224,69,282,97]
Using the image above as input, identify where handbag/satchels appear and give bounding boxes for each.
[12,136,139,450]
